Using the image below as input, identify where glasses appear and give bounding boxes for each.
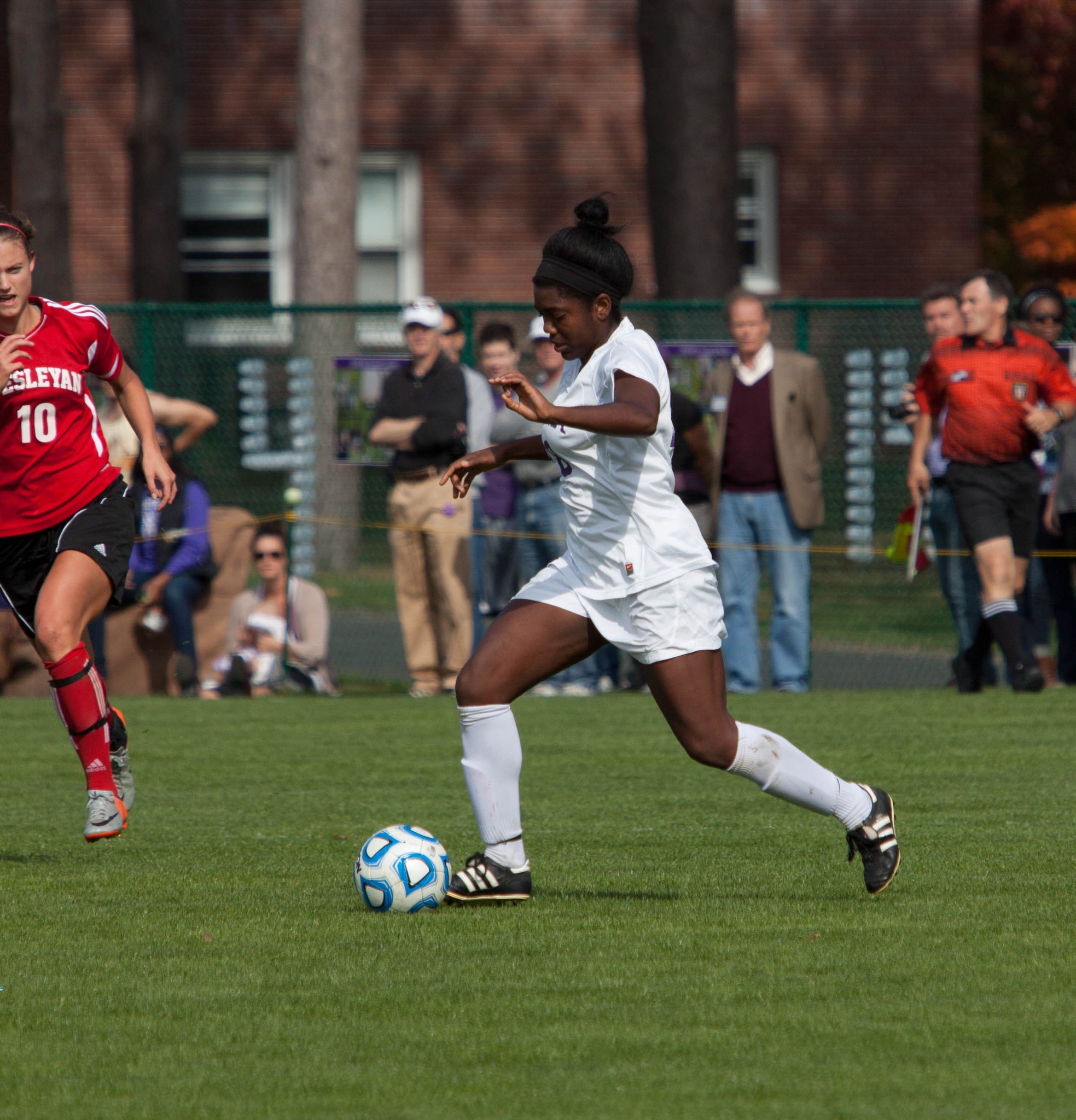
[254,551,281,560]
[1033,311,1062,324]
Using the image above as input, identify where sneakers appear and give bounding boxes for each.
[847,782,900,893]
[447,852,532,902]
[109,708,135,812]
[83,787,125,838]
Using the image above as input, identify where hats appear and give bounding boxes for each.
[400,296,441,327]
[527,317,553,338]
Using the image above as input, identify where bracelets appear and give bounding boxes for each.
[1052,405,1066,426]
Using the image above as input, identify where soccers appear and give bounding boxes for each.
[354,825,451,915]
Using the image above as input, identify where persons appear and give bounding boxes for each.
[702,293,831,696]
[442,194,902,902]
[86,346,218,699]
[897,272,1076,697]
[366,293,715,698]
[0,208,179,843]
[198,523,336,699]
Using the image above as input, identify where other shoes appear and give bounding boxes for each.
[1010,667,1044,692]
[956,664,979,692]
